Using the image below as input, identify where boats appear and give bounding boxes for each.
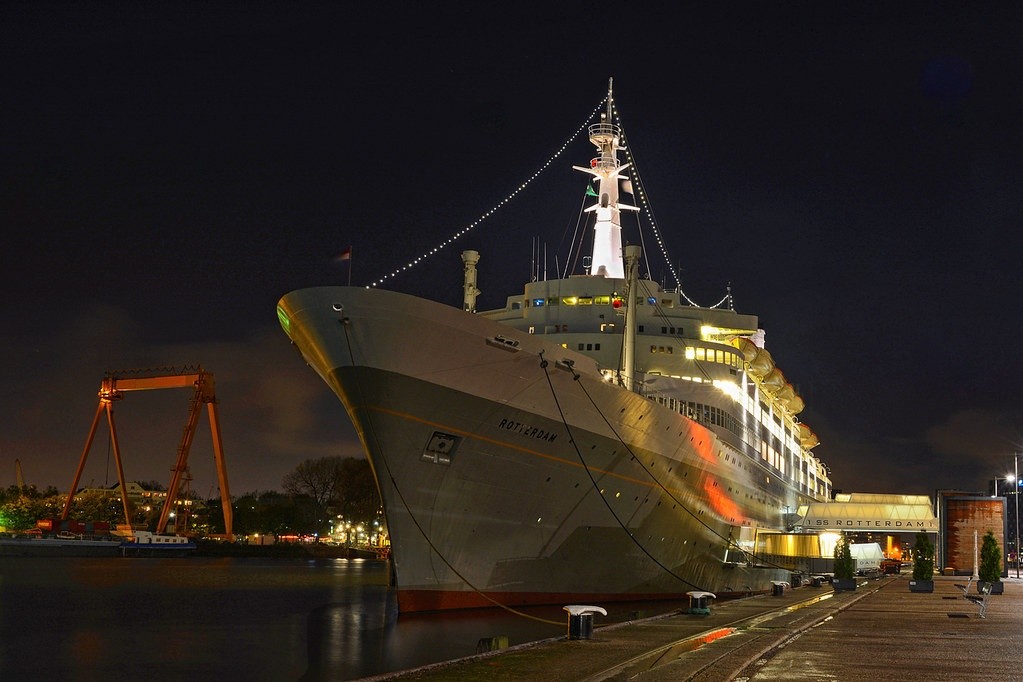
[56,531,75,539]
[117,531,201,558]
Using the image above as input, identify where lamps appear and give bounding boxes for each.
[806,451,831,474]
[757,374,801,425]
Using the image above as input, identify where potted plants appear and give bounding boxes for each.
[908,531,934,593]
[833,531,856,592]
[978,527,1004,596]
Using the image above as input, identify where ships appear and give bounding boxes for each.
[275,78,837,615]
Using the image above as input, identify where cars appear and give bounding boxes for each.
[1008,547,1023,568]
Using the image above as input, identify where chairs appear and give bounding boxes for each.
[954,576,974,598]
[964,582,993,618]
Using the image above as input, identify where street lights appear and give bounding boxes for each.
[995,475,1015,497]
